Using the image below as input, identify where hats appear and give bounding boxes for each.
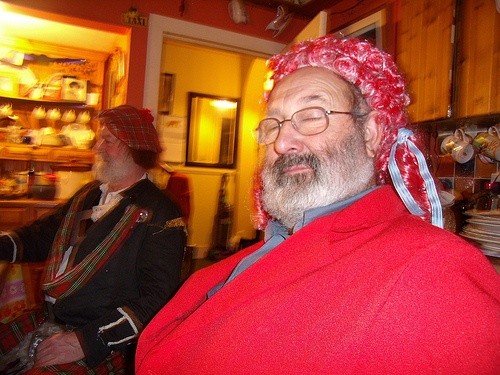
[98,104,161,150]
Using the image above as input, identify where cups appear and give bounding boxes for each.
[472,126,500,162]
[441,128,474,164]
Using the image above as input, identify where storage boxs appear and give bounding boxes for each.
[62,76,87,102]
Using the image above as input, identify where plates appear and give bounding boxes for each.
[458,210,500,258]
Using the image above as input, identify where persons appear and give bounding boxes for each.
[0,105,191,375]
[136,32,500,375]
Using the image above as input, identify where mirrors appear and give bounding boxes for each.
[186,92,240,169]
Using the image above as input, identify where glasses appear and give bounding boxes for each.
[255,106,351,145]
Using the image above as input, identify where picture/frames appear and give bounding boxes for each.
[158,71,176,114]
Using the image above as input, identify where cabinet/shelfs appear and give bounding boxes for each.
[411,113,500,208]
[0,205,60,302]
